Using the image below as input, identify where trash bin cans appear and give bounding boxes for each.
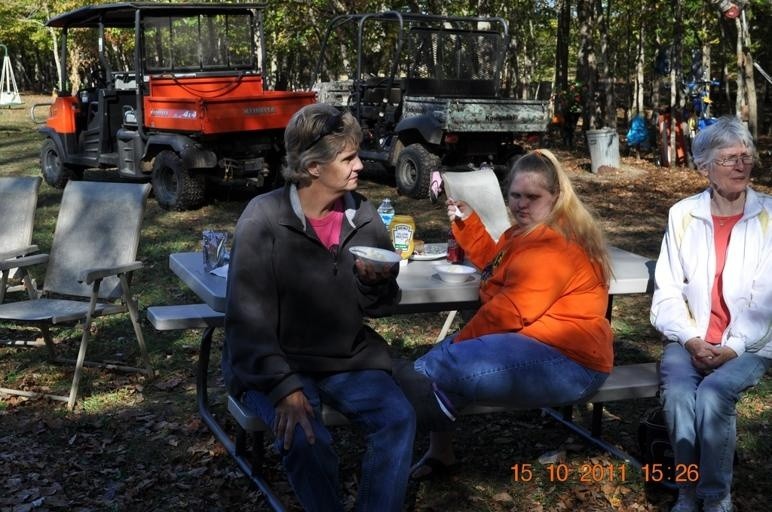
[585,128,620,174]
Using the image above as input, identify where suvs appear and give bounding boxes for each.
[29,1,318,212]
[288,11,550,200]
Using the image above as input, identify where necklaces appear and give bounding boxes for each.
[714,200,744,226]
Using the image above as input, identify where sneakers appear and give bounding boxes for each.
[671,488,699,512]
[703,493,732,512]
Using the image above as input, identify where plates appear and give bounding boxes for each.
[409,244,446,261]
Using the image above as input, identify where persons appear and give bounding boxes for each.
[221,105,417,510]
[410,148,615,482]
[648,119,772,511]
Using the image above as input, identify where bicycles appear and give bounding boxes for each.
[627,78,720,171]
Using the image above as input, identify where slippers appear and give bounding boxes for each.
[411,458,443,480]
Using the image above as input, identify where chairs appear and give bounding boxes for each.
[0,176,42,346]
[0,180,151,414]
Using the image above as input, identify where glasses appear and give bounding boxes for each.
[305,106,345,151]
[714,155,754,167]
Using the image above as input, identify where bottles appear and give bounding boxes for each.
[376,197,395,233]
[389,215,415,260]
[446,229,465,265]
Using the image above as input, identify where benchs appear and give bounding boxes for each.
[228,363,659,472]
[146,302,225,405]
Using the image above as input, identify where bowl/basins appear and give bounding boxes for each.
[348,245,403,273]
[432,264,478,284]
